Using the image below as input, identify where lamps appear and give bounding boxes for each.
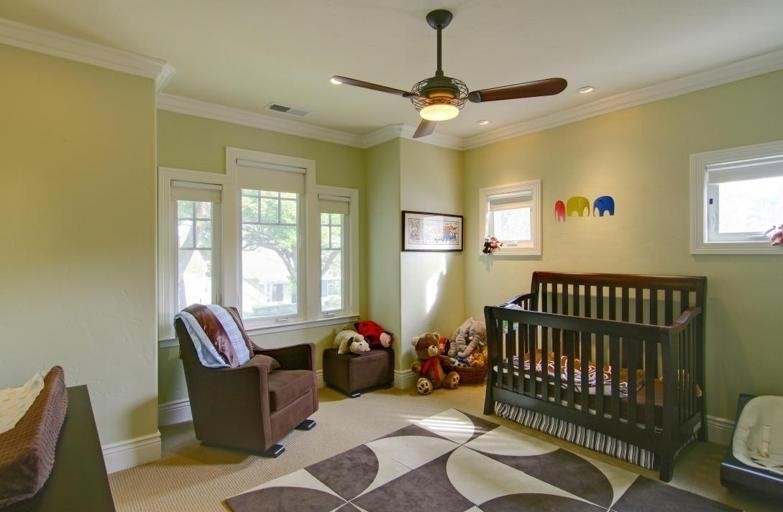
[415,98,462,121]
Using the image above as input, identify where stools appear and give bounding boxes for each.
[322,347,393,399]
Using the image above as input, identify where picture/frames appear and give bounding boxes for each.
[400,209,464,253]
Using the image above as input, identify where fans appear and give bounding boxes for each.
[331,8,567,138]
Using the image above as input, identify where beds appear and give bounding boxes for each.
[481,270,711,483]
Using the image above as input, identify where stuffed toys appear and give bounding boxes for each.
[333,329,371,355]
[353,318,394,348]
[410,317,488,396]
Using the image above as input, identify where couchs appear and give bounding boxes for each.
[173,303,318,458]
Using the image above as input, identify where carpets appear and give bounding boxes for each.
[224,406,747,512]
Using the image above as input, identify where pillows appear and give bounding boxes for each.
[239,353,279,376]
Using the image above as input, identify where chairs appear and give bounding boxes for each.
[719,391,782,504]
